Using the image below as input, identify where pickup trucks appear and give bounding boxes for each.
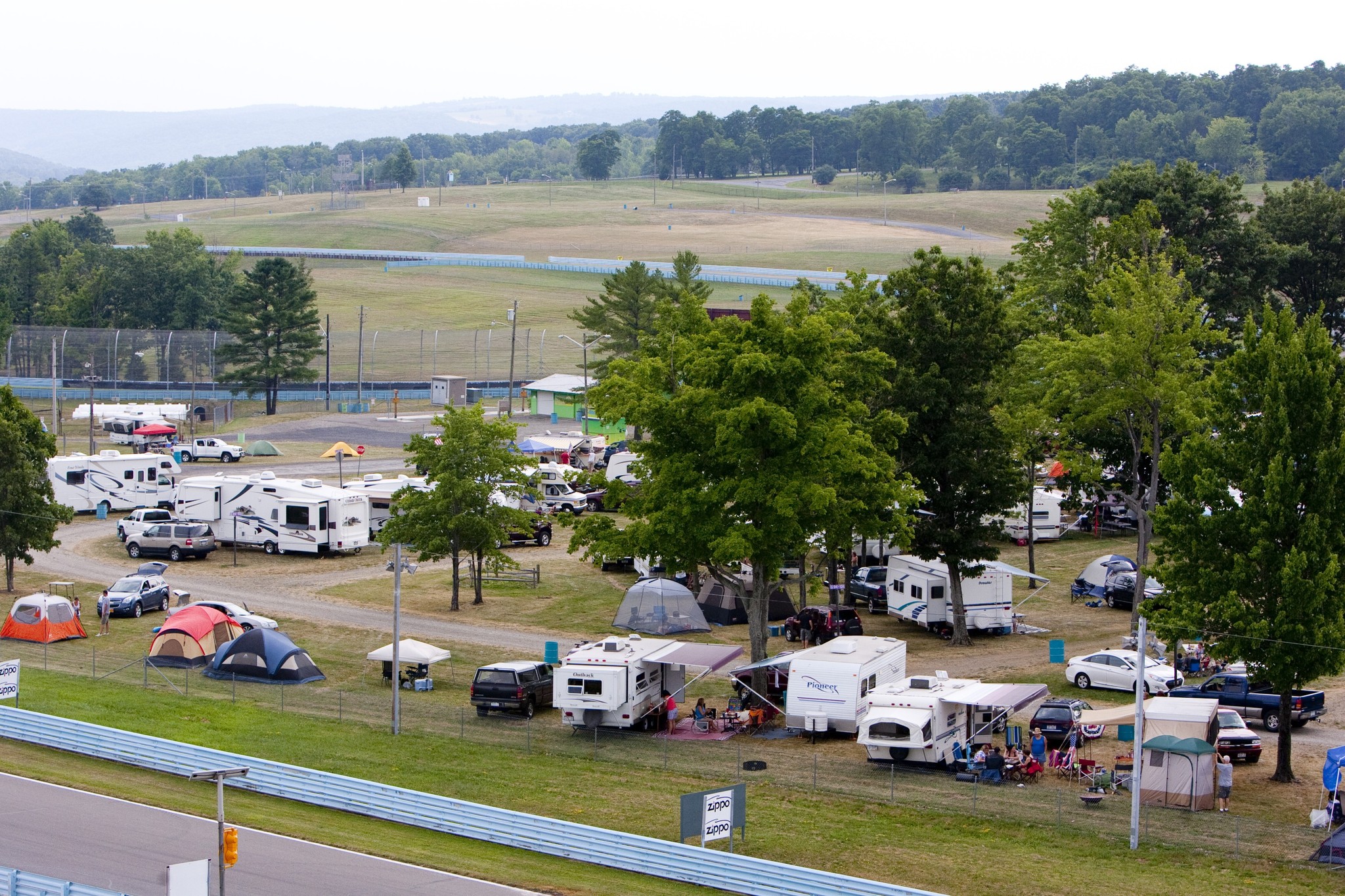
[171,437,245,463]
[1167,670,1328,732]
[118,508,178,542]
[849,565,888,615]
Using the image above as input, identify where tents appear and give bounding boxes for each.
[145,606,457,691]
[1044,462,1069,485]
[0,593,88,644]
[1319,745,1345,832]
[611,573,799,636]
[131,425,363,456]
[507,439,557,463]
[1080,495,1152,538]
[1073,554,1137,598]
[1072,697,1217,812]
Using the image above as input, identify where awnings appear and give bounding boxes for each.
[640,642,813,718]
[980,561,1050,612]
[939,683,1050,762]
[530,436,586,452]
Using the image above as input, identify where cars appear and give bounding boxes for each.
[1100,560,1175,611]
[1065,649,1185,694]
[730,650,797,704]
[1226,656,1269,672]
[591,529,634,570]
[165,589,279,634]
[1215,708,1262,763]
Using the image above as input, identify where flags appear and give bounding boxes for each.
[569,443,573,454]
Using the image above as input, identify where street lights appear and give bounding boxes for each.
[559,334,612,435]
[385,543,418,734]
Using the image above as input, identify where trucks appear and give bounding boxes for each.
[29,449,182,513]
[342,472,444,535]
[175,471,371,555]
[473,461,589,515]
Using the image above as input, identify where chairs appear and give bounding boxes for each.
[1114,764,1133,792]
[143,582,148,588]
[132,435,185,454]
[966,743,1133,792]
[628,606,669,627]
[1337,791,1345,825]
[381,660,429,685]
[691,701,779,737]
[1005,726,1027,752]
[1172,656,1221,679]
[1071,578,1095,604]
[534,505,556,516]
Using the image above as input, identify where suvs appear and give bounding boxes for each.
[784,604,863,646]
[124,521,216,562]
[1028,698,1100,748]
[96,562,170,618]
[586,481,645,513]
[490,515,554,549]
[470,661,554,720]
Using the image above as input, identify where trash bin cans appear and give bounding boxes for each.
[1118,725,1134,742]
[551,413,558,424]
[544,641,559,664]
[783,691,787,714]
[1050,640,1064,664]
[575,411,583,422]
[237,432,245,443]
[96,503,108,520]
[174,451,182,464]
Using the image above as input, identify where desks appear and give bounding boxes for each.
[635,614,651,626]
[825,567,853,581]
[668,615,690,631]
[642,708,668,733]
[1012,612,1029,631]
[1006,759,1020,780]
[48,581,75,599]
[1113,756,1133,776]
[720,711,742,734]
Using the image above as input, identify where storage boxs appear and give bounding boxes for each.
[415,679,434,692]
[767,624,785,637]
[1122,777,1133,792]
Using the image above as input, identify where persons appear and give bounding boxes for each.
[71,590,110,636]
[974,729,1047,780]
[1077,507,1107,532]
[1169,641,1228,669]
[1214,752,1232,812]
[852,552,859,575]
[661,690,776,735]
[560,447,629,475]
[794,608,818,649]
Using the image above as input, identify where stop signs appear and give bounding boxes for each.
[357,445,365,455]
